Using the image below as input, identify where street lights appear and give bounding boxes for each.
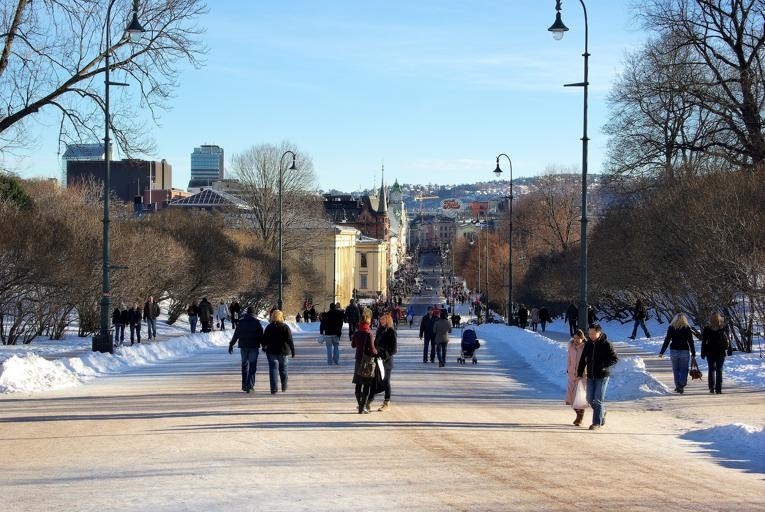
[547,0,591,338]
[469,233,481,295]
[397,255,412,294]
[492,152,515,327]
[474,207,490,323]
[276,149,298,310]
[332,206,348,306]
[89,0,147,353]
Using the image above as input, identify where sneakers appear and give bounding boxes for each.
[602,412,607,425]
[589,424,600,430]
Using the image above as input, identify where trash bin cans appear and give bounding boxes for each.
[91,328,115,354]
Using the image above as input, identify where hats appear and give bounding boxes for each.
[270,310,283,324]
[247,307,255,314]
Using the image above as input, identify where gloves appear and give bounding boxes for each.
[228,347,233,354]
[262,347,265,352]
[290,351,294,358]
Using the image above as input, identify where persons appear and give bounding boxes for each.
[356,312,397,412]
[260,309,296,395]
[565,328,587,425]
[577,321,619,430]
[389,244,487,368]
[112,294,389,348]
[228,305,264,394]
[351,315,380,414]
[658,313,697,395]
[507,298,601,340]
[627,298,651,339]
[701,312,730,394]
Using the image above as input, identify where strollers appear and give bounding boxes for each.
[455,324,481,366]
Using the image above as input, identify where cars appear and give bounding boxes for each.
[467,313,502,324]
[425,285,433,291]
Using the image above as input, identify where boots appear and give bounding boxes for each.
[355,392,363,414]
[378,400,390,411]
[575,410,584,426]
[361,393,369,414]
[573,411,580,424]
[366,399,373,412]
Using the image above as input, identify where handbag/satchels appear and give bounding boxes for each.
[374,355,385,383]
[690,358,702,381]
[356,333,376,378]
[216,320,219,328]
